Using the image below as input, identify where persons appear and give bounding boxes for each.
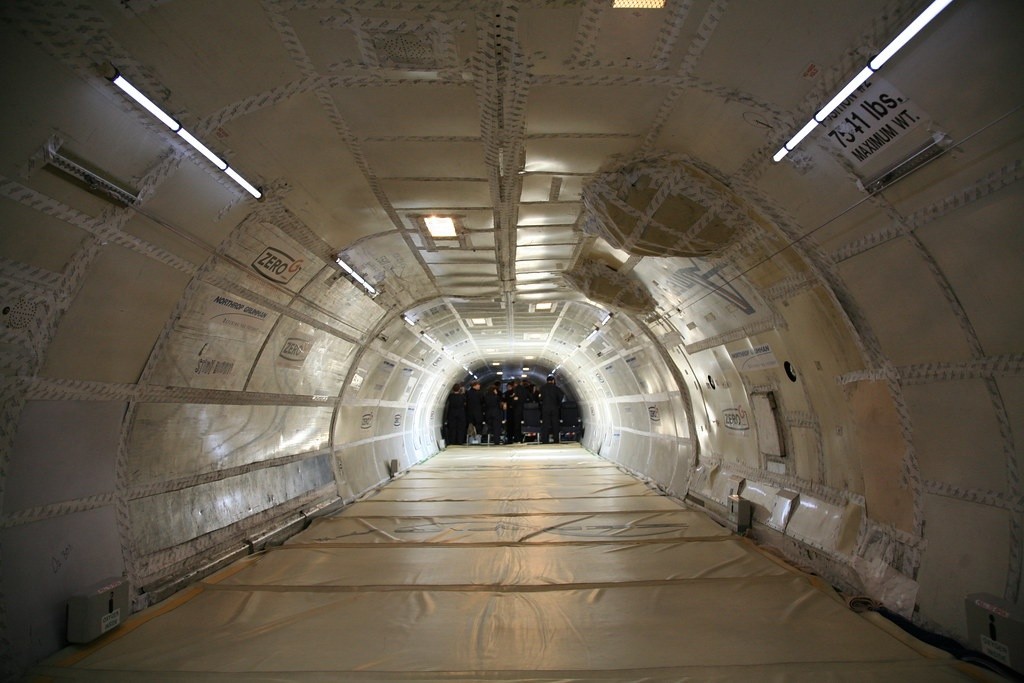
[538,376,563,444]
[445,377,541,446]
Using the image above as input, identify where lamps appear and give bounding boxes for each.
[331,255,376,295]
[442,348,459,364]
[563,345,580,362]
[420,331,435,344]
[102,63,265,203]
[585,327,599,341]
[773,0,953,163]
[401,314,415,327]
[602,313,613,326]
